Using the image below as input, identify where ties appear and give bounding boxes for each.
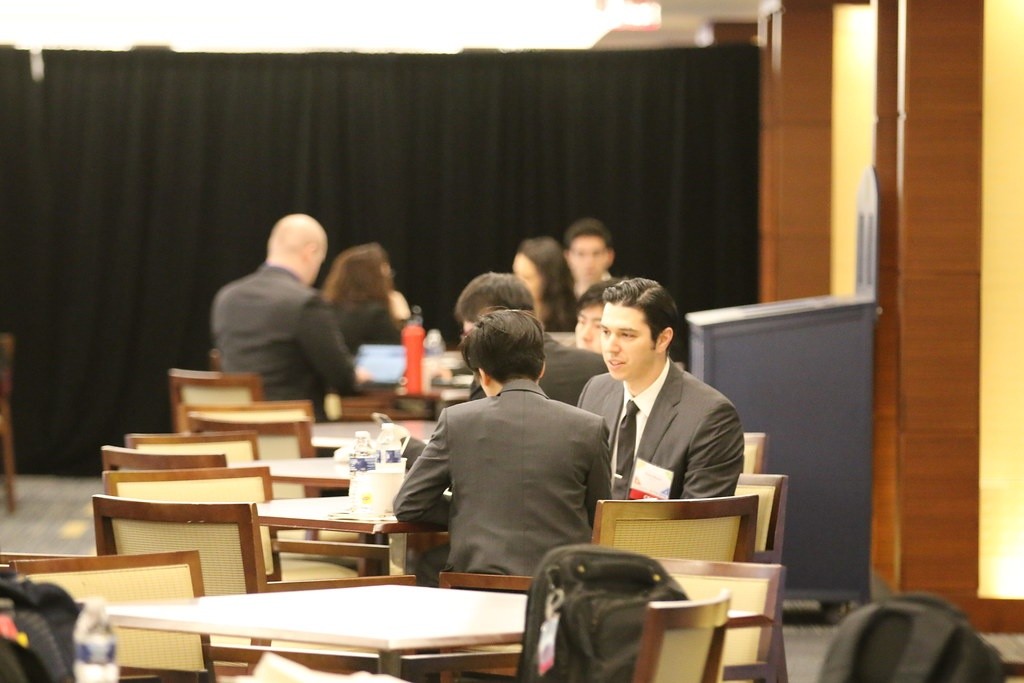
[616,401,640,498]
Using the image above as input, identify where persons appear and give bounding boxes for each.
[382,272,608,470]
[393,310,611,592]
[324,243,412,355]
[572,279,626,353]
[513,235,579,332]
[576,277,745,499]
[210,213,368,496]
[562,218,617,299]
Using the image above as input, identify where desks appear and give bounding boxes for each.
[102,584,528,683]
[227,457,354,487]
[309,419,440,450]
[258,497,452,578]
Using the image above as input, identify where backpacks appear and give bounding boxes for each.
[817,592,1004,683]
[0,572,84,683]
[517,544,689,683]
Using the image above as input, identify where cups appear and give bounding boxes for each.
[374,458,408,515]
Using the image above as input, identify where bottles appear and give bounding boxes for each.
[73,597,120,683]
[373,423,403,463]
[422,328,446,397]
[348,431,373,518]
[402,319,426,398]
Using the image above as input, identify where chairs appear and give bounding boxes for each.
[440,572,537,683]
[651,556,786,683]
[592,494,760,562]
[733,474,791,683]
[0,334,362,683]
[740,431,767,473]
[631,591,732,683]
